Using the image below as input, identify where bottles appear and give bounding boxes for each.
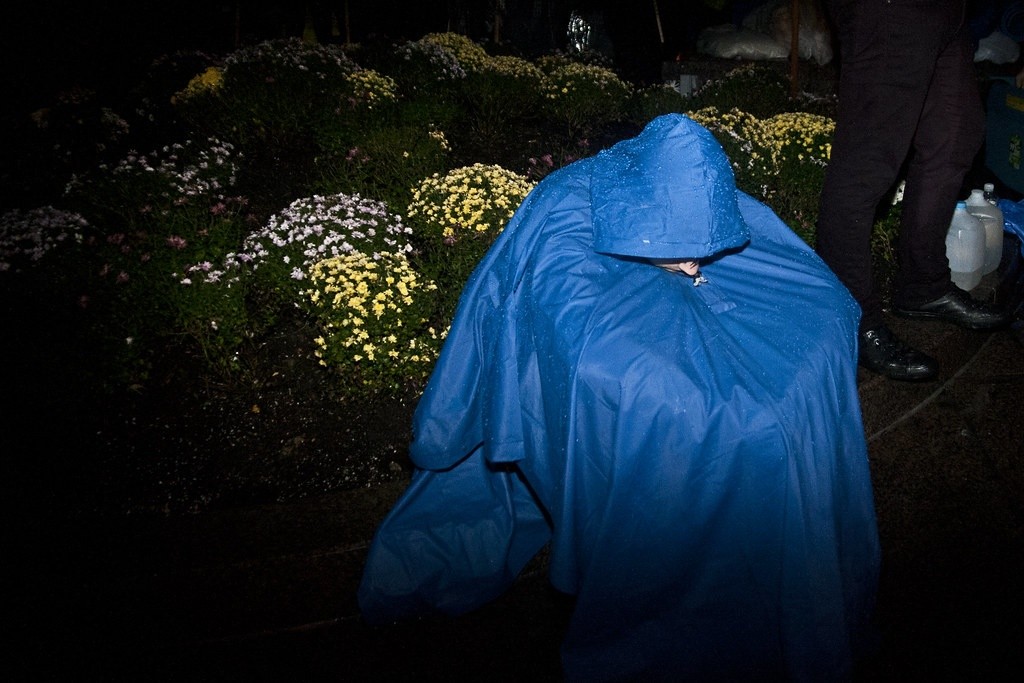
[965,189,1003,278]
[946,200,986,292]
[983,183,1001,210]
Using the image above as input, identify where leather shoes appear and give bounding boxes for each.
[894,290,1012,330]
[853,322,938,382]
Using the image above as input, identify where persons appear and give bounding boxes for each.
[350,114,882,682]
[816,0,1017,381]
[973,31,1023,202]
